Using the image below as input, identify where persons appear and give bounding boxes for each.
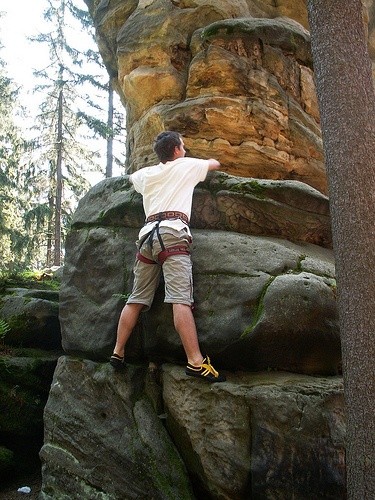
[108,130,223,380]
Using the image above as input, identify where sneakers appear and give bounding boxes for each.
[186,355,227,383]
[110,354,127,368]
